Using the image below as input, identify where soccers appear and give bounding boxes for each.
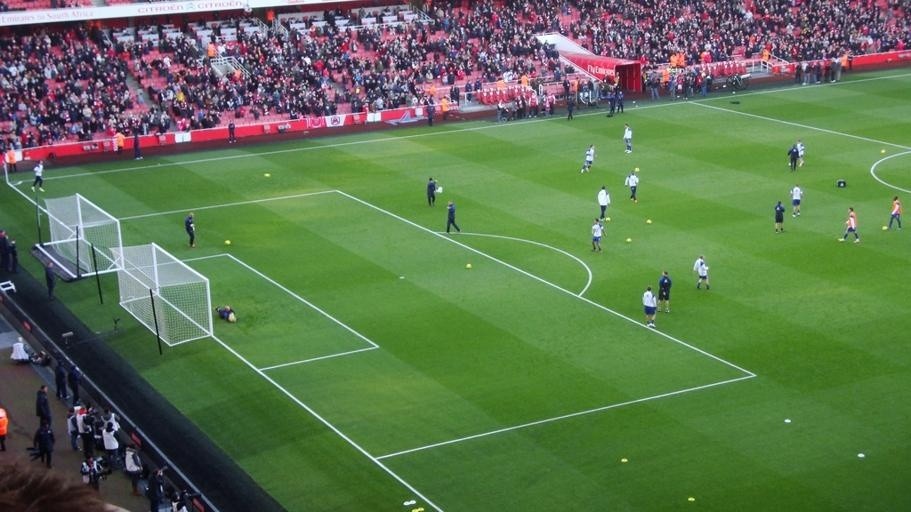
[626,238,631,243]
[264,173,270,179]
[466,263,471,269]
[225,239,231,246]
[604,218,611,221]
[646,220,652,224]
[635,167,640,172]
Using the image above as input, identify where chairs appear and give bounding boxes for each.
[0,1,910,173]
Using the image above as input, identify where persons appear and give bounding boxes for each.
[790,184,803,218]
[642,287,657,328]
[838,207,860,243]
[0,230,19,273]
[214,306,236,323]
[31,160,45,192]
[694,256,710,290]
[598,185,611,221]
[657,271,672,313]
[624,170,639,203]
[185,213,195,247]
[591,218,607,252]
[774,201,785,234]
[888,196,902,230]
[427,178,438,207]
[0,337,202,511]
[1,0,911,173]
[45,262,56,301]
[447,200,460,234]
